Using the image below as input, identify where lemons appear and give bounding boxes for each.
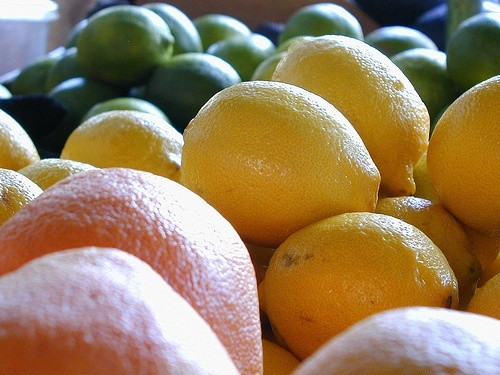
[0,3,500,375]
